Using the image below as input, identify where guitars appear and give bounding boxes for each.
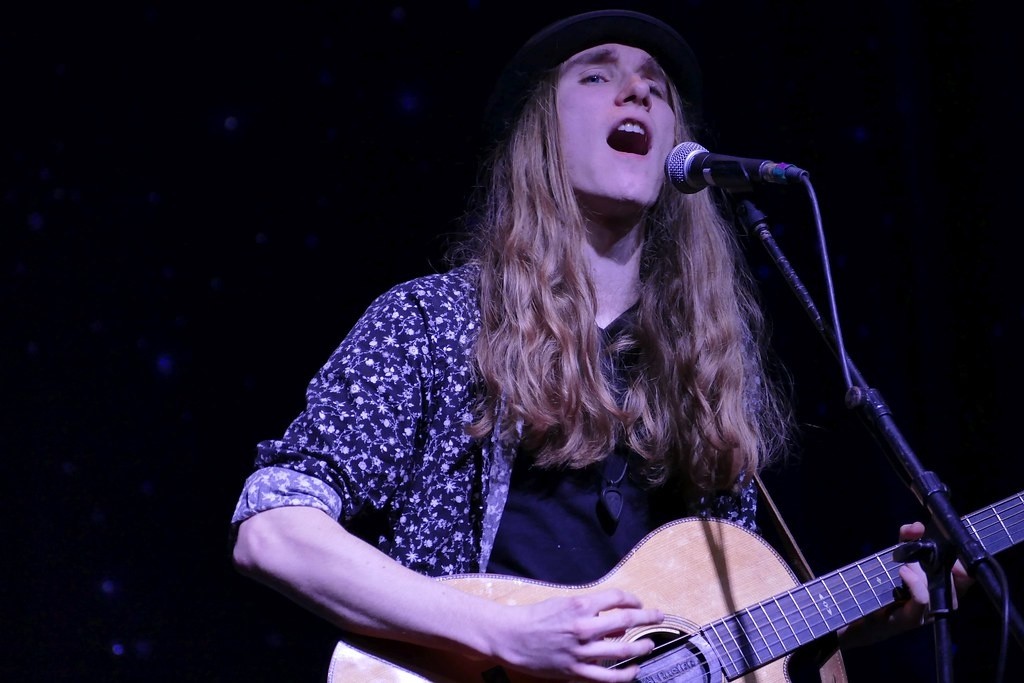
[326,490,1023,683]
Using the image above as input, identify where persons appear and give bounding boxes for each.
[231,11,969,683]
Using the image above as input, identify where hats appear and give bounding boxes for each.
[494,7,704,138]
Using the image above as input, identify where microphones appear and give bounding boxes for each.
[664,141,809,194]
